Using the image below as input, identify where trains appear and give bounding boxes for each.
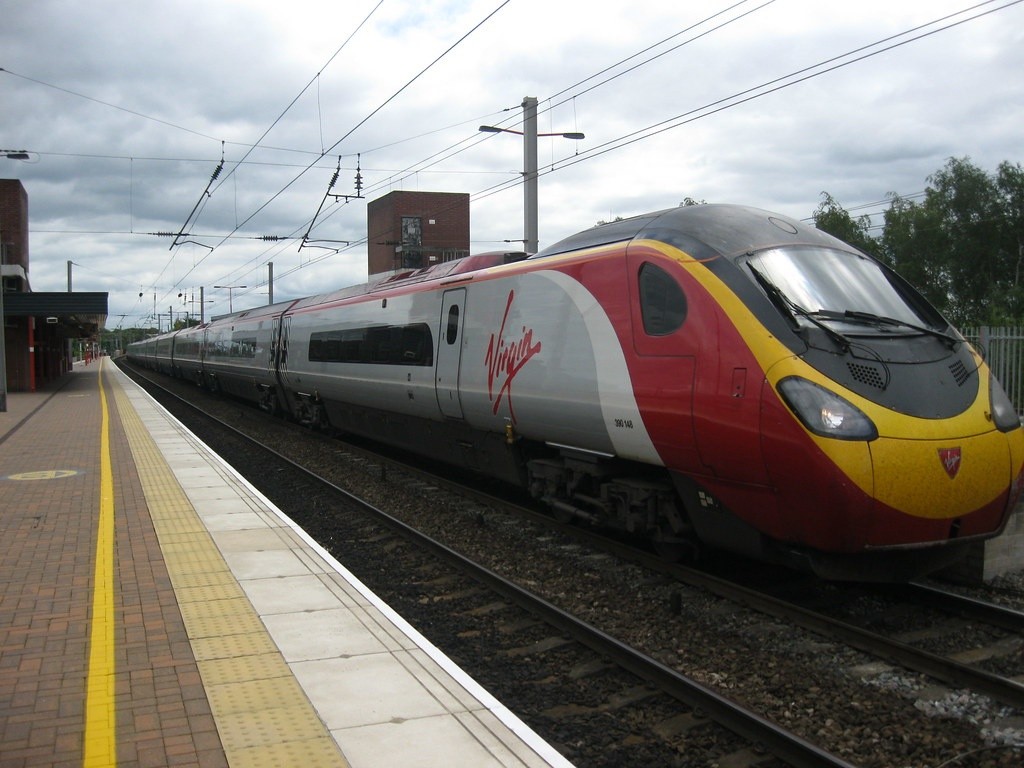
[122,205,1024,602]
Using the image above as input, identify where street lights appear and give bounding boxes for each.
[214,286,247,315]
[188,300,214,325]
[475,123,584,253]
